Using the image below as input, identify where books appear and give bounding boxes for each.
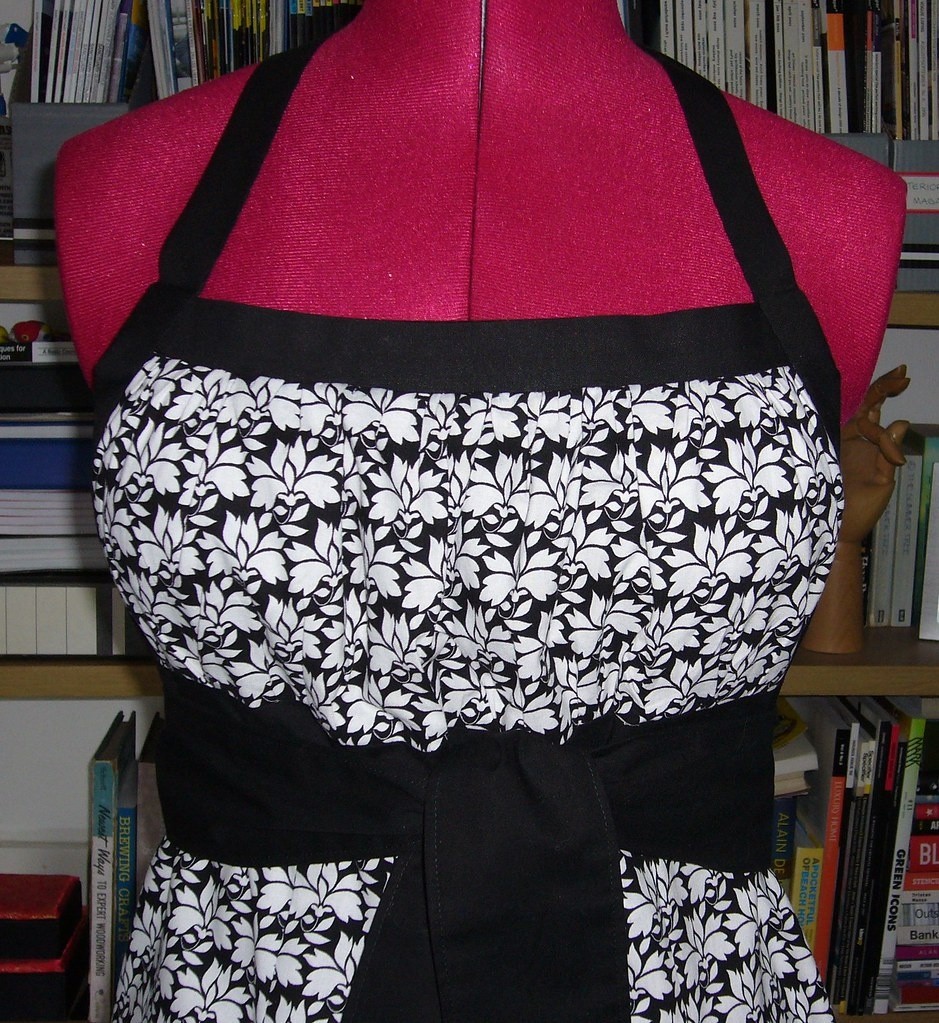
[1,697,939,1023]
[0,0,939,661]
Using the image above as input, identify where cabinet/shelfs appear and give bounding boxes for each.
[0,0,939,1023]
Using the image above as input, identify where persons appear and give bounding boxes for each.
[57,3,909,1023]
[803,363,910,654]
